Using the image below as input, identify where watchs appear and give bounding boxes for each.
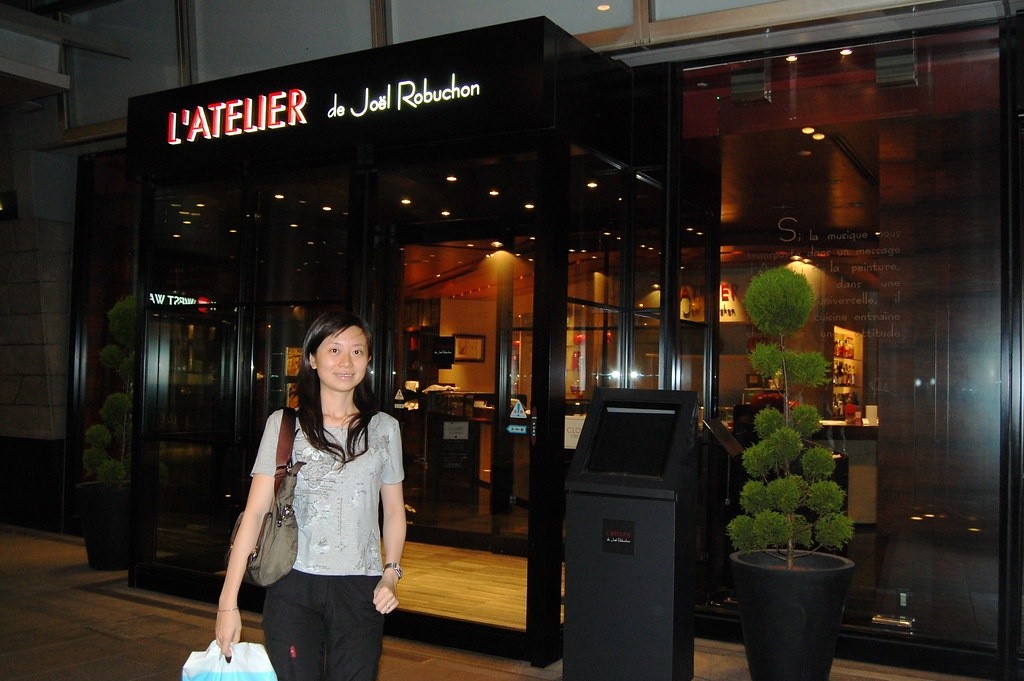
[383,563,402,579]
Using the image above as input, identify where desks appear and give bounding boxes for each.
[805,425,880,532]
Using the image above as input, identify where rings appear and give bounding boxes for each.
[386,605,390,609]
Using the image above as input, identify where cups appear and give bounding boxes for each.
[846,404,856,420]
[866,405,878,419]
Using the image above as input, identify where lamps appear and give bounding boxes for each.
[404,369,420,390]
[789,244,813,263]
[585,167,599,189]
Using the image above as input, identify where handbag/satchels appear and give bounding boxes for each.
[226,406,307,586]
[181,640,277,681]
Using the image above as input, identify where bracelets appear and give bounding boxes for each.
[218,608,238,612]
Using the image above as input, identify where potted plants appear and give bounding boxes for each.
[729,267,855,681]
[72,295,158,572]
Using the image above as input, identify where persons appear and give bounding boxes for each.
[217,312,407,681]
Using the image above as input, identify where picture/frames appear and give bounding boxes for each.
[452,333,485,363]
